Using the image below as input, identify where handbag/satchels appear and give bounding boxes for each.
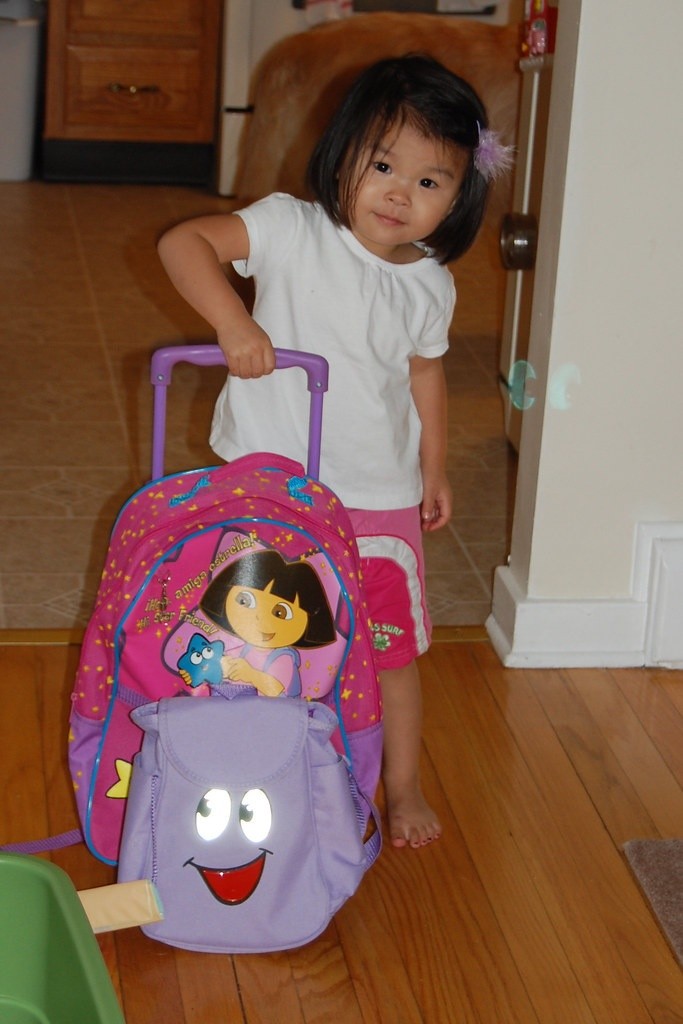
[117,697,363,953]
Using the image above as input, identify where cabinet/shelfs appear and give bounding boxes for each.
[38,0,222,188]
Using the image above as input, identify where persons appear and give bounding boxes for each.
[154,51,491,856]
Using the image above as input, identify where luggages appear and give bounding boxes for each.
[66,341,391,866]
